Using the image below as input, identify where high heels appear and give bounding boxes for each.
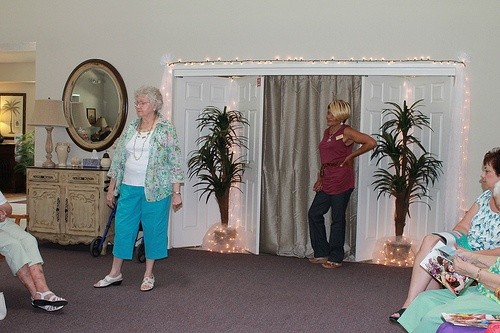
[93,274,123,288]
[140,273,154,292]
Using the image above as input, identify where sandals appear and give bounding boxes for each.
[33,291,68,306]
[30,297,64,311]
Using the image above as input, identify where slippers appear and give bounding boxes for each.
[388,307,407,323]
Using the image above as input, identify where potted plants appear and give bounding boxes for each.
[370,98,444,261]
[186,106,254,247]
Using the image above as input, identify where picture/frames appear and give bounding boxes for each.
[0,93,26,140]
[86,108,97,125]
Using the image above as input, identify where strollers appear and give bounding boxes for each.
[89,178,146,264]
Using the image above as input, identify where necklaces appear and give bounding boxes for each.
[133,114,156,160]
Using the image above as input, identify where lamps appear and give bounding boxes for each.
[27,97,69,167]
[93,116,108,130]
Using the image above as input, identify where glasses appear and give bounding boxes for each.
[135,100,150,107]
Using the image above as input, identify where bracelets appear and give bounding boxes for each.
[173,191,182,196]
[475,268,483,283]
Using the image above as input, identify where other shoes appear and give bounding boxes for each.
[323,261,342,268]
[309,257,328,263]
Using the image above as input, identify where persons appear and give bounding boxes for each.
[397,178,500,333]
[0,192,69,312]
[429,256,469,296]
[388,146,500,325]
[307,99,377,269]
[92,86,187,291]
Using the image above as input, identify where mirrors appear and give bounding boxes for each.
[62,59,128,152]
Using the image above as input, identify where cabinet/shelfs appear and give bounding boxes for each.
[25,168,115,256]
